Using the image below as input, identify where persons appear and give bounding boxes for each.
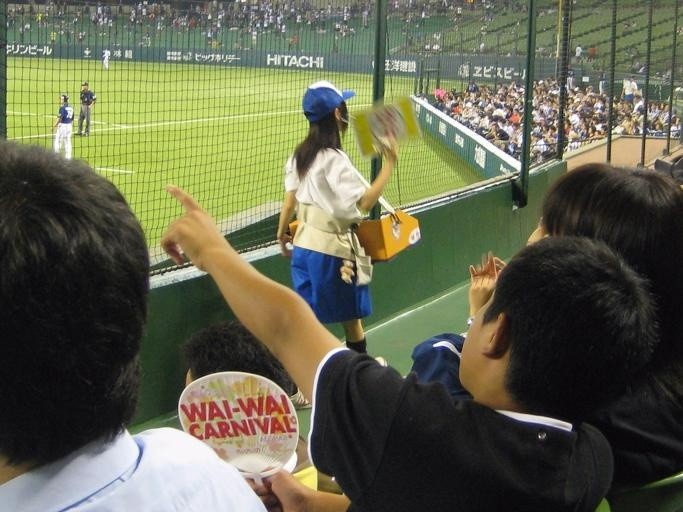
[2,3,683,512]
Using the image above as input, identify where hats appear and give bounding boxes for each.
[80,83,89,89]
[654,158,683,187]
[302,81,355,122]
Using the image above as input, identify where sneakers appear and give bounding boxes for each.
[290,388,310,410]
[73,130,88,138]
[375,356,388,366]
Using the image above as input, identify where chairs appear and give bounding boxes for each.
[606,471,683,512]
